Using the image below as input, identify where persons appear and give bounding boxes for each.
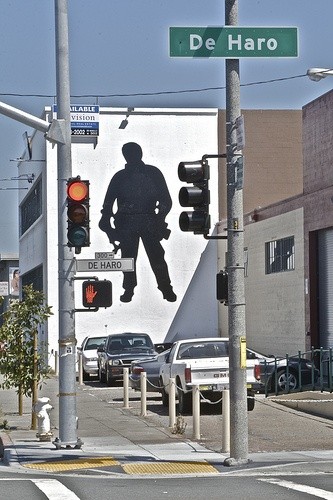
[12,270,19,295]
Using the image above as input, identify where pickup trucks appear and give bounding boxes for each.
[158,338,262,416]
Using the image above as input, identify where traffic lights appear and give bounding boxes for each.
[177,161,209,234]
[67,179,91,247]
[82,280,112,307]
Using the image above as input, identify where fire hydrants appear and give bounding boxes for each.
[31,395,55,441]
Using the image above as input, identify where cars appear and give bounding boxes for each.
[129,346,320,395]
[153,343,173,352]
[76,332,111,381]
[98,332,155,387]
